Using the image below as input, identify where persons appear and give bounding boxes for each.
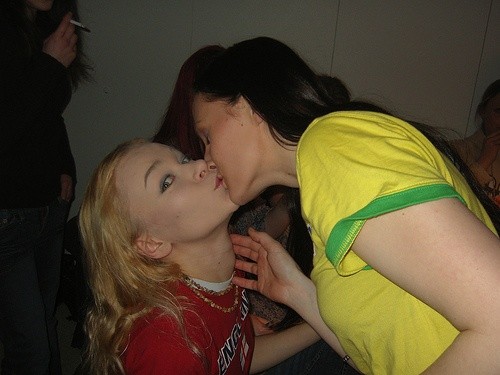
[0,0,500,375]
[153,36,500,375]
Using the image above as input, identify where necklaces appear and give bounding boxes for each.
[184,279,239,313]
[184,271,236,296]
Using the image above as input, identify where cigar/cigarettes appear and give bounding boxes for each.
[70,19,85,28]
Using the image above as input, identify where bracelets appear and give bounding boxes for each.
[343,355,350,361]
